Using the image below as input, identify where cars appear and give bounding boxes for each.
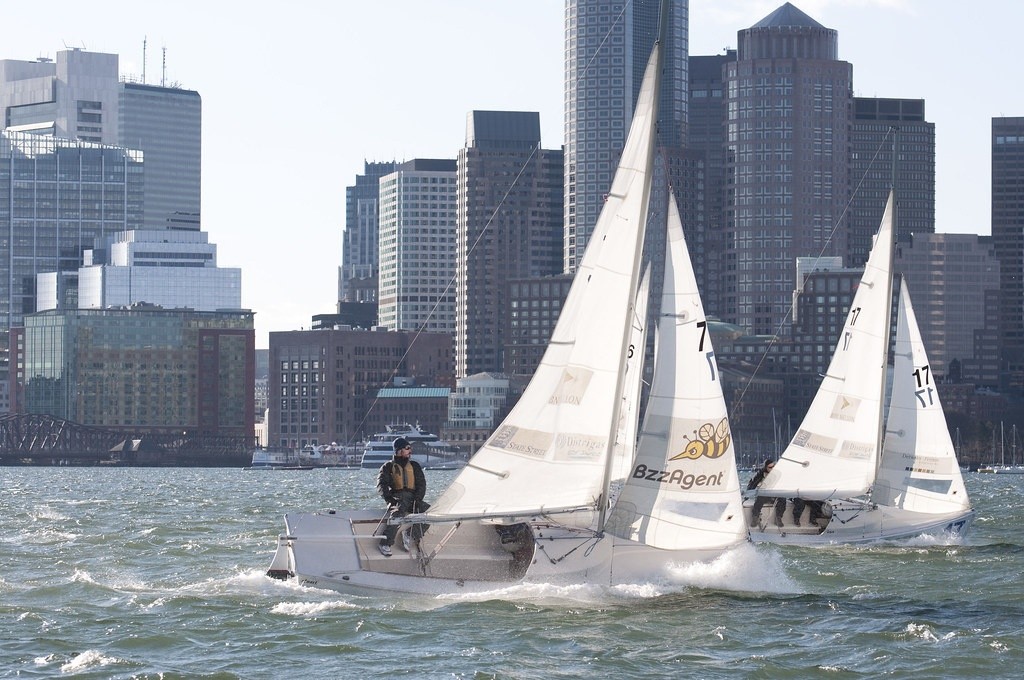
[303,441,364,453]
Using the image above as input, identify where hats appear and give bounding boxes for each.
[764,459,774,466]
[393,437,412,451]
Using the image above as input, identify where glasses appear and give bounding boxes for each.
[768,464,775,469]
[402,446,412,450]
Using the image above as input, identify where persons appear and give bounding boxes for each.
[744,459,786,527]
[788,498,818,527]
[377,438,431,556]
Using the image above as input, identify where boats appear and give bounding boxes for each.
[242,447,315,470]
[360,421,469,469]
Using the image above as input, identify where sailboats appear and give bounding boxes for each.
[953,421,1024,474]
[742,125,977,546]
[263,0,746,604]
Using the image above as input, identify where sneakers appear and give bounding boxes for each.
[378,544,393,557]
[809,517,819,527]
[750,515,762,527]
[774,516,785,527]
[400,530,411,552]
[793,517,801,526]
